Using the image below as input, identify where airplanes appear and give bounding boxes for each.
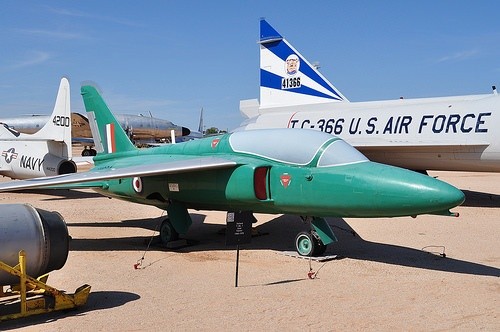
[0,18,500,258]
